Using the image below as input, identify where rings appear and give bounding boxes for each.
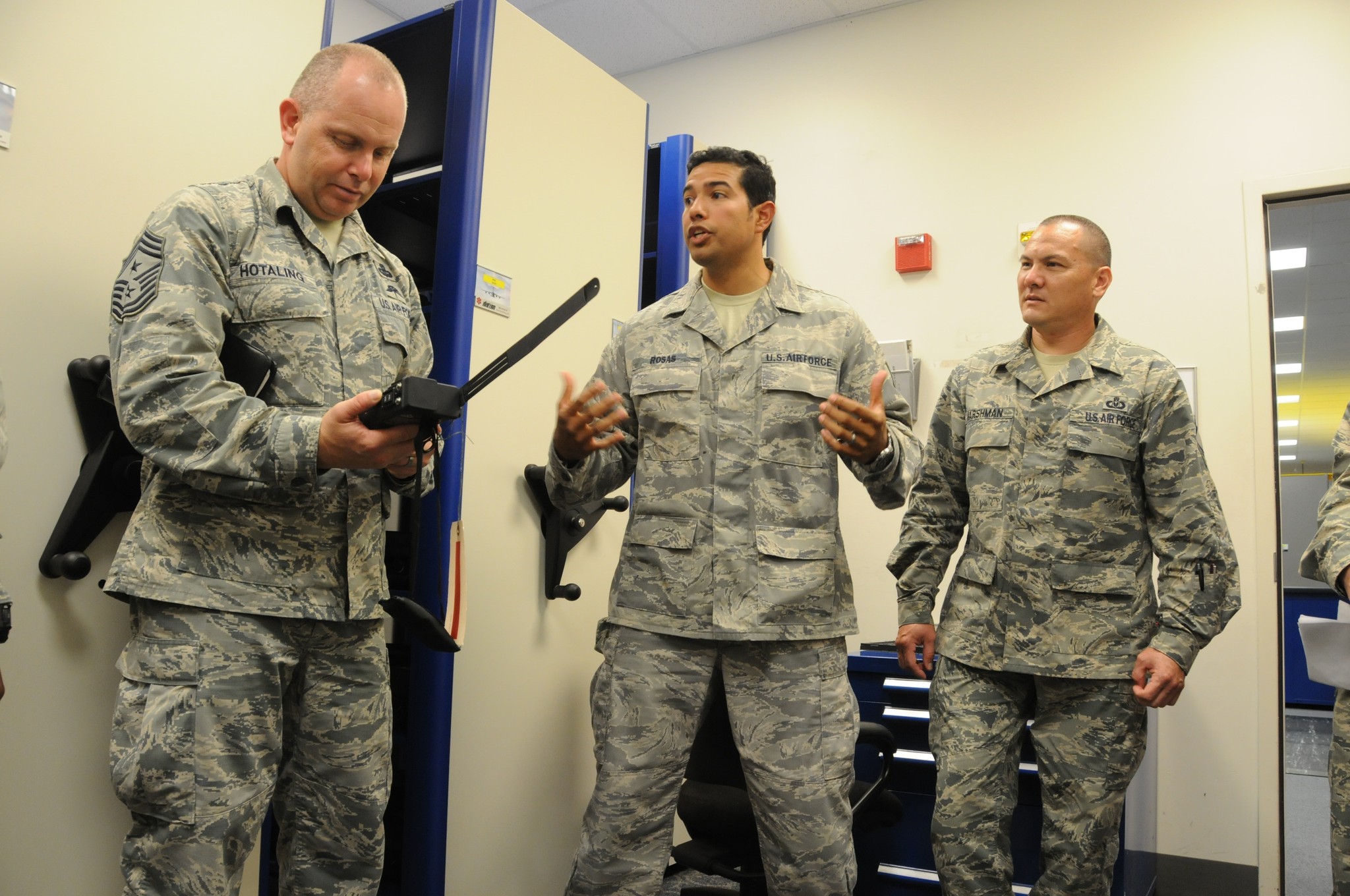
[850,433,857,446]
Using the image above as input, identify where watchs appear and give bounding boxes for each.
[858,424,895,473]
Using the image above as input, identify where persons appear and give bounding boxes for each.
[541,147,925,896]
[102,42,447,895]
[886,213,1241,896]
[1299,400,1350,896]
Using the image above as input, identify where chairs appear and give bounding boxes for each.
[670,661,905,896]
